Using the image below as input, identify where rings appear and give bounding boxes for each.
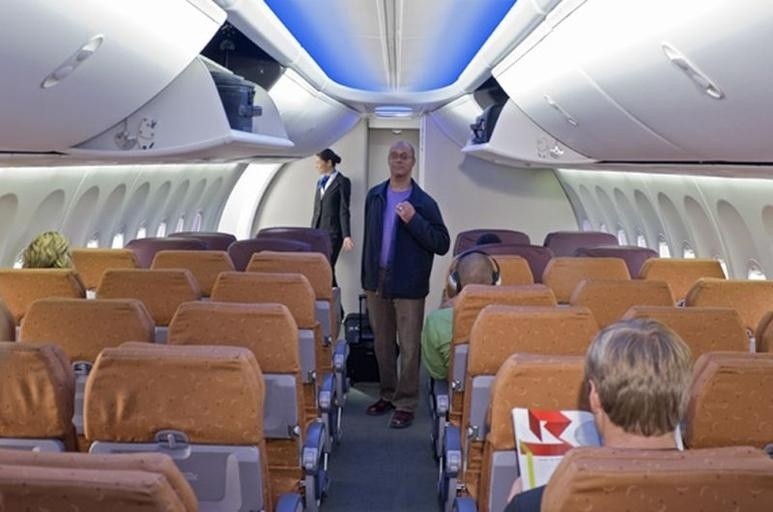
[399,206,404,211]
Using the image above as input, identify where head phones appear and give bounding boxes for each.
[446,248,502,296]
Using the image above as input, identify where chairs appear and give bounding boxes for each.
[449,231,535,255]
[615,302,750,359]
[225,237,314,275]
[165,298,336,511]
[541,255,631,303]
[682,274,771,340]
[441,300,603,508]
[147,246,238,278]
[635,255,732,303]
[447,242,558,284]
[564,276,675,329]
[443,349,605,510]
[252,228,335,264]
[0,335,77,447]
[123,235,205,268]
[479,254,537,291]
[208,268,346,451]
[542,230,624,260]
[90,265,202,346]
[683,348,772,450]
[79,338,306,512]
[64,245,142,289]
[165,230,238,254]
[14,293,163,352]
[0,445,203,512]
[0,262,87,328]
[573,243,663,281]
[536,442,771,510]
[240,247,348,417]
[426,279,561,451]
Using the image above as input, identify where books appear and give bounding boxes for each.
[512,406,684,493]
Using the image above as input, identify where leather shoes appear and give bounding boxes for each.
[390,411,413,428]
[366,399,395,416]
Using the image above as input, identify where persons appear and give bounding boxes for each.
[503,317,697,512]
[0,297,17,342]
[360,141,450,429]
[311,149,354,319]
[476,233,502,246]
[421,251,494,380]
[21,231,96,299]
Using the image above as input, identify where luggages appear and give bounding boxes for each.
[344,295,399,386]
